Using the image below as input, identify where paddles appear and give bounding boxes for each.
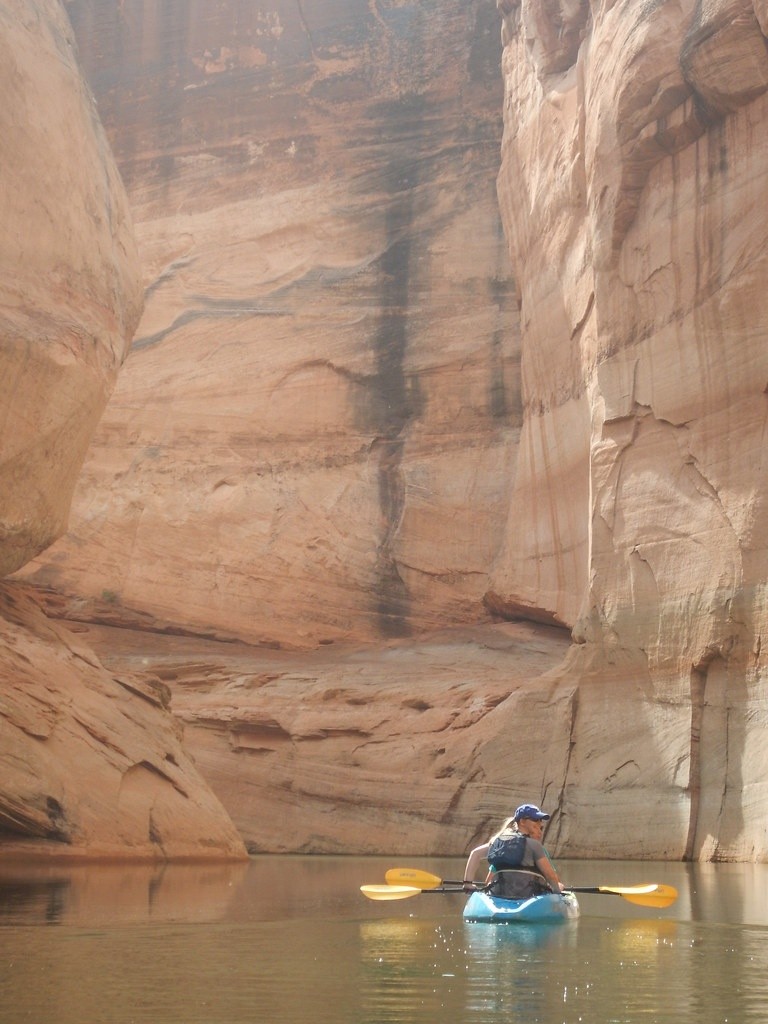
[361,883,677,909]
[385,867,651,891]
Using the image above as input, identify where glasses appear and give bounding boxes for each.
[522,817,542,823]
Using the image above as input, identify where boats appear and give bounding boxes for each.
[463,891,581,922]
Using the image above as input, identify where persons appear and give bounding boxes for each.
[485,828,558,885]
[463,804,564,894]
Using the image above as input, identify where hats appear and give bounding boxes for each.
[514,804,550,822]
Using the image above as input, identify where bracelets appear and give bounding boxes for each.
[463,881,472,884]
